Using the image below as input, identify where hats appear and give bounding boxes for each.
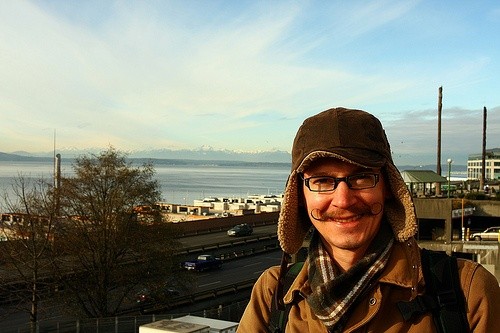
[276,106,419,255]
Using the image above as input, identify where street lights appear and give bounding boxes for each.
[447,158,453,197]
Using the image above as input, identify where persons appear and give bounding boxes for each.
[235,107,500,333]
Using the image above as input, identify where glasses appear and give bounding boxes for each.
[298,167,382,193]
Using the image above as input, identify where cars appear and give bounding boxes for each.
[470,226,500,241]
[227,223,253,236]
[184,255,220,273]
